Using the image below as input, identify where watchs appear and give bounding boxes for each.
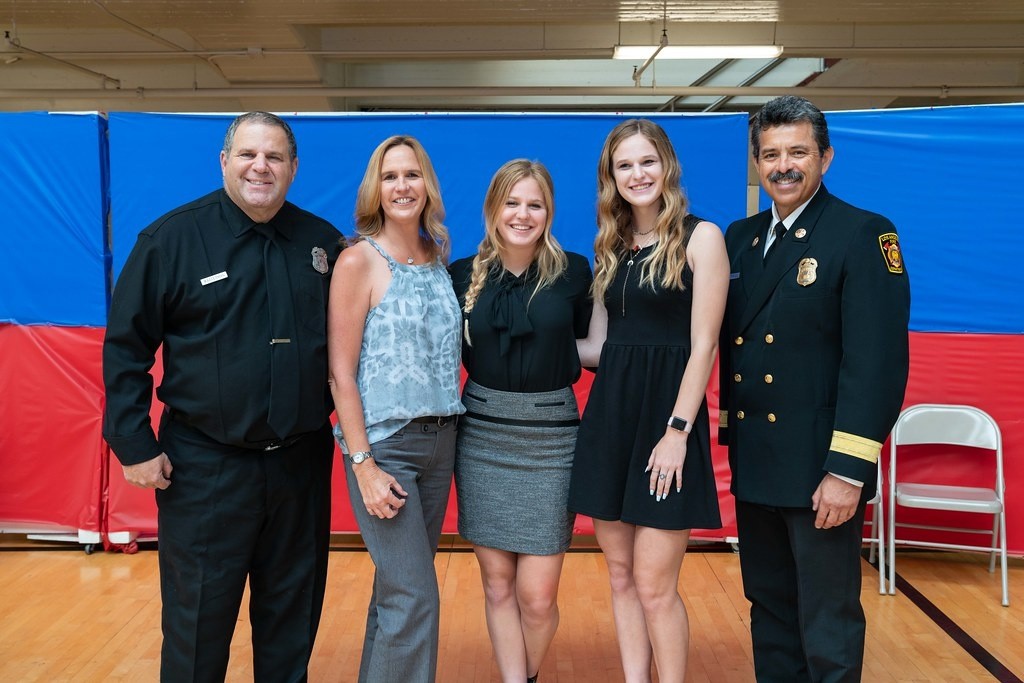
[349,450,375,464]
[667,416,693,434]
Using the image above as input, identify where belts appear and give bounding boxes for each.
[412,416,453,428]
[164,404,334,452]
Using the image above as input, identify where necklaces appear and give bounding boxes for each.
[626,220,656,267]
[382,231,421,264]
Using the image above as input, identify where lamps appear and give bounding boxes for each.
[613,45,783,59]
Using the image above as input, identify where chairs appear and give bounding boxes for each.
[862,449,887,595]
[888,402,1010,607]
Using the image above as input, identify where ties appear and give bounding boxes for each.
[251,223,299,440]
[764,221,787,260]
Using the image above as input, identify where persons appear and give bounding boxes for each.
[446,158,600,683]
[102,111,349,683]
[718,94,912,683]
[573,118,734,683]
[328,135,468,683]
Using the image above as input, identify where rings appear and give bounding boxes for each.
[652,469,660,474]
[658,474,667,480]
